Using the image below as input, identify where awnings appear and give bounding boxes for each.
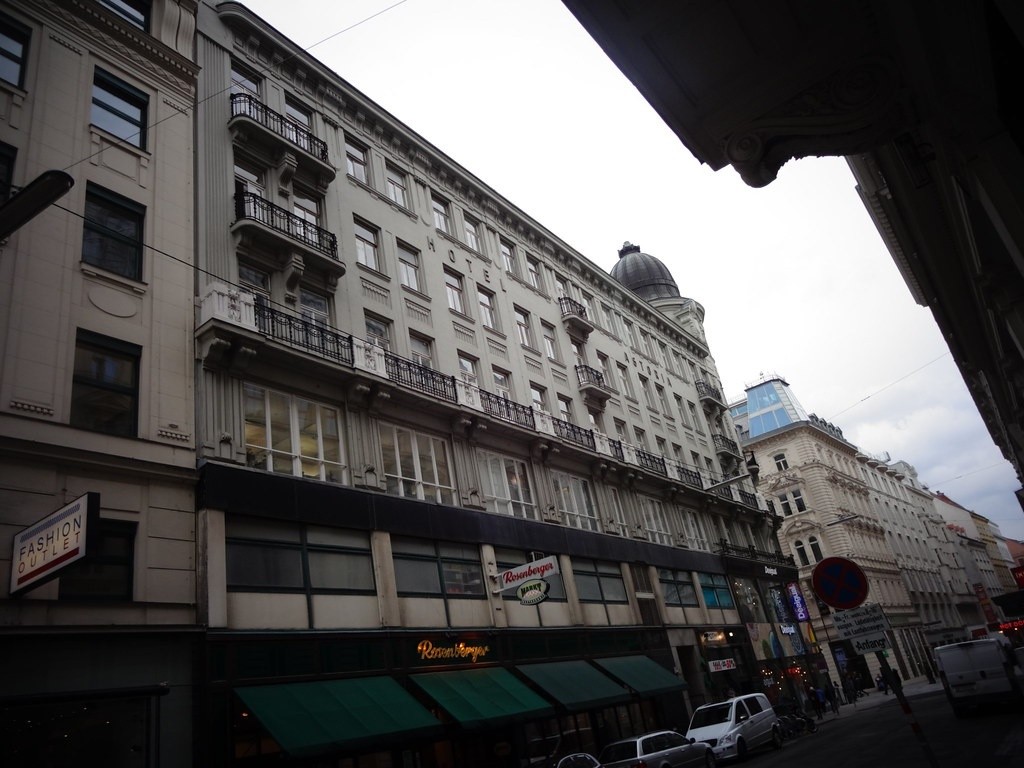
[231,655,688,768]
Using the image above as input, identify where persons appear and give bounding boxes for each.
[726,688,735,699]
[825,684,839,715]
[875,666,903,695]
[922,660,935,684]
[809,687,823,720]
[816,685,826,713]
[846,671,869,700]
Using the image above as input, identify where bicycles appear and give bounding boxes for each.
[777,716,819,741]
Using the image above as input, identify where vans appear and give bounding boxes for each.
[685,692,782,763]
[933,638,1020,709]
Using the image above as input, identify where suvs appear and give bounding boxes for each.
[602,730,716,768]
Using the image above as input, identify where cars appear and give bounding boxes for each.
[545,753,601,768]
